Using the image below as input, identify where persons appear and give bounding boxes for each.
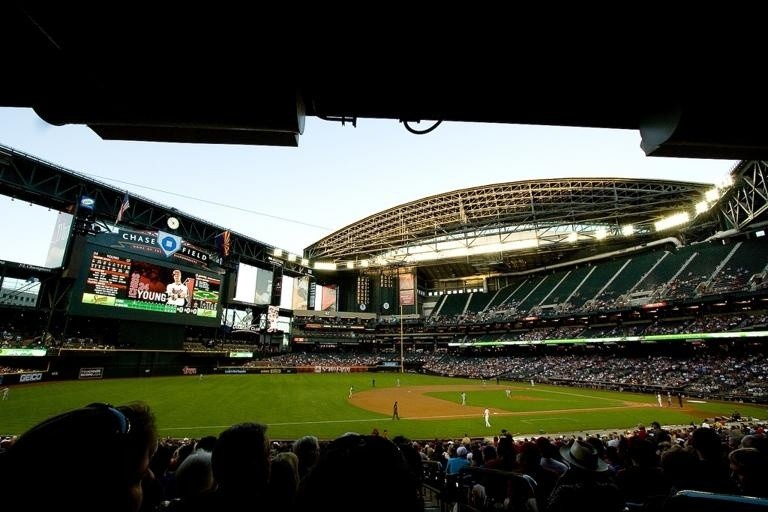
[1,400,767,512]
[2,386,9,400]
[0,323,116,374]
[165,269,188,305]
[182,265,767,402]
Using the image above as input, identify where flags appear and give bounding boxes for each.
[114,191,130,225]
[223,229,230,259]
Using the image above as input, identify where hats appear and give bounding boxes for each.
[559,437,609,472]
